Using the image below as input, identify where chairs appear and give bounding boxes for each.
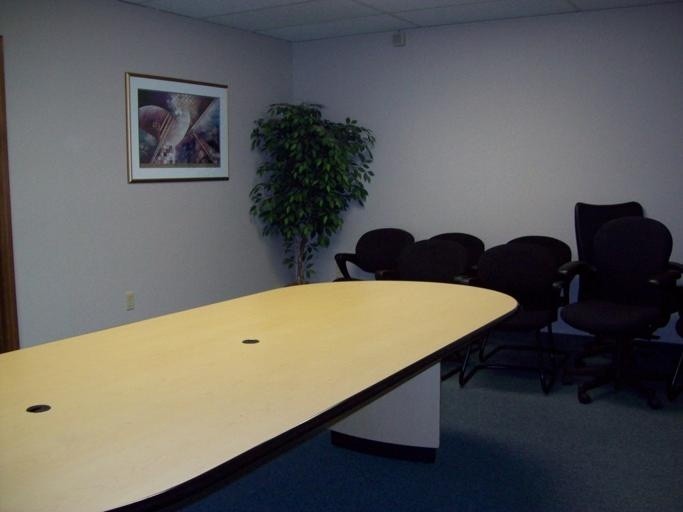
[392,230,488,386]
[455,234,574,400]
[573,203,646,293]
[335,225,415,287]
[559,214,680,407]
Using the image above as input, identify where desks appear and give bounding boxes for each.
[0,281,523,512]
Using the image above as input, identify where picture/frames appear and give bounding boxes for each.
[125,72,229,183]
